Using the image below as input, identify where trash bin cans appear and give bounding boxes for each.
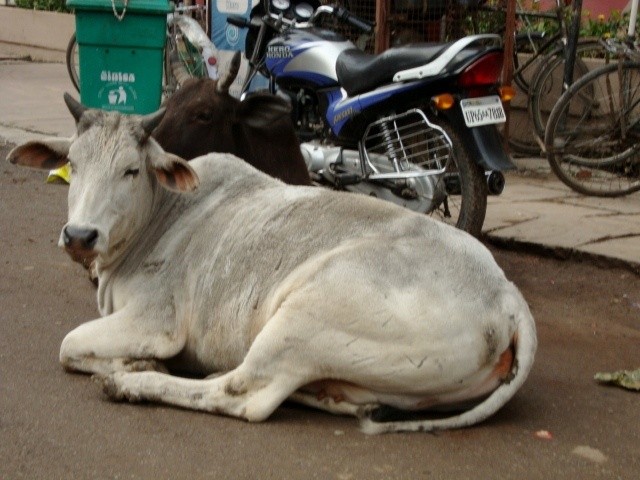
[65,0,175,116]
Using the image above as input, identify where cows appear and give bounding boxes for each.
[150,47,312,186]
[5,92,539,436]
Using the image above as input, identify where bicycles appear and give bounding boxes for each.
[528,38,640,166]
[66,0,219,99]
[451,0,594,154]
[544,35,640,197]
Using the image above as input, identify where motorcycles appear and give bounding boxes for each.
[227,0,517,238]
